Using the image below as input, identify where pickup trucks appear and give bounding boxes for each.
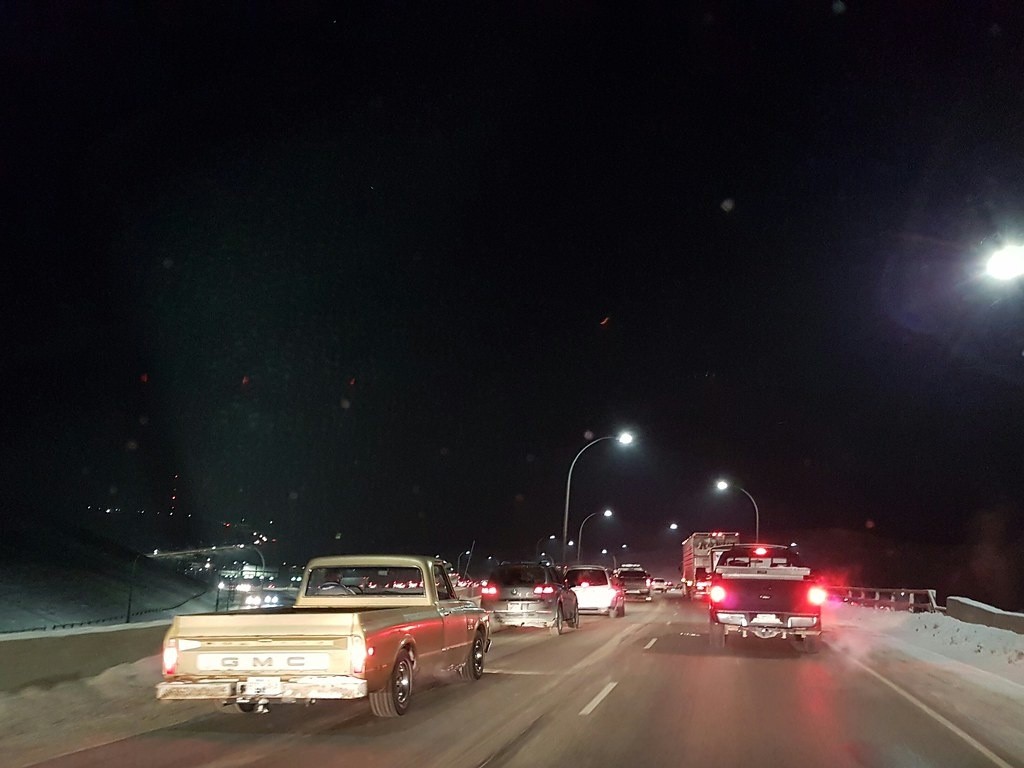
[705,543,823,646]
[157,555,493,719]
[613,564,652,602]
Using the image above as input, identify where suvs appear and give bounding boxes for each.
[480,560,580,636]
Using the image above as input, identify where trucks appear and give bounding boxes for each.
[678,532,740,599]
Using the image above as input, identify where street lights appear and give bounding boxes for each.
[535,535,556,563]
[717,480,759,544]
[562,434,633,567]
[577,509,612,565]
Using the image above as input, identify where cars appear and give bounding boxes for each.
[217,575,299,611]
[562,563,626,618]
[651,577,668,593]
